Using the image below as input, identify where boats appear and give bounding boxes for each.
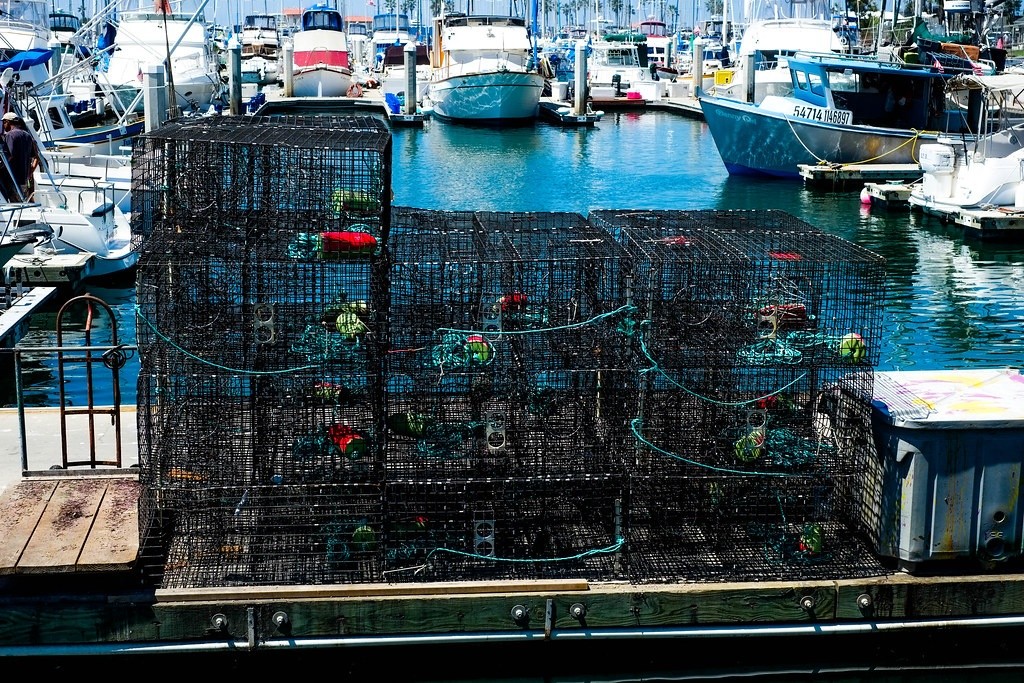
[0,0,1024,290]
[906,69,1024,223]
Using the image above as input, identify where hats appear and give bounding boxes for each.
[1,112,19,121]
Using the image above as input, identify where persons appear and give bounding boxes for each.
[0,112,40,227]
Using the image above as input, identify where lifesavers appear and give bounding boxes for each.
[346,82,364,97]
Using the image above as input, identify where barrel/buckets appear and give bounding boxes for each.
[924,171,952,199]
[550,82,567,100]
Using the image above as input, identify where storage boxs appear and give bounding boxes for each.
[841,368,1024,573]
